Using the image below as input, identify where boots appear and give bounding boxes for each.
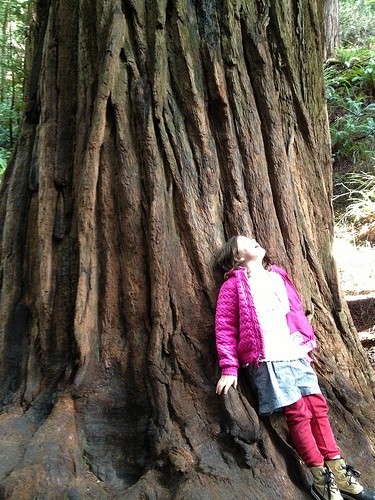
[308,459,374,500]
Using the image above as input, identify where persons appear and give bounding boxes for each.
[214,234,375,500]
